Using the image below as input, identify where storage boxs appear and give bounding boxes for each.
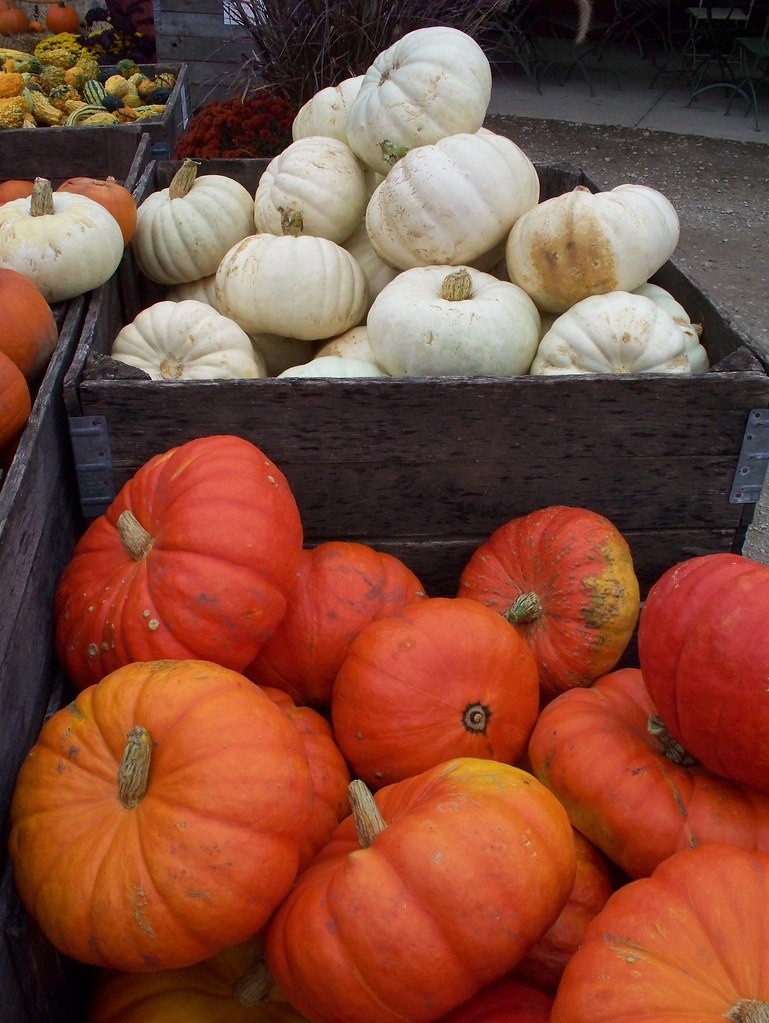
[1,60,768,1022]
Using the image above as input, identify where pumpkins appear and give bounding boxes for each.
[0,0,707,442]
[7,432,769,1023]
